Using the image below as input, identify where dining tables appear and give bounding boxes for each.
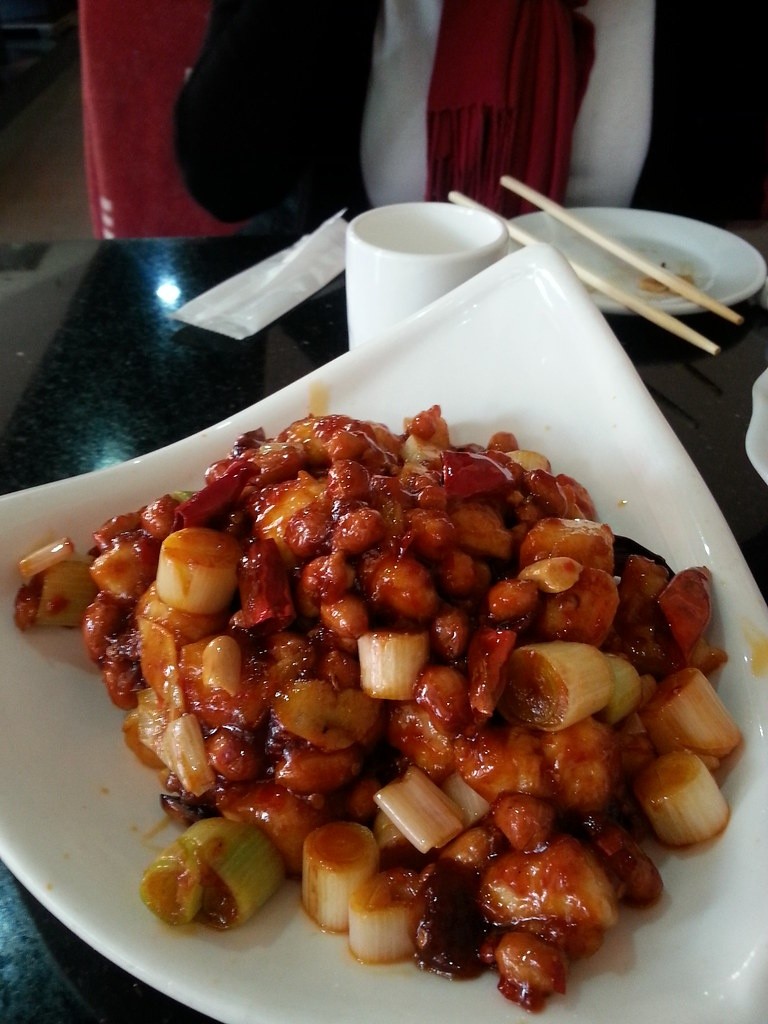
[0,237,768,1024]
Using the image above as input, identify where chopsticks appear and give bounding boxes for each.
[446,175,743,356]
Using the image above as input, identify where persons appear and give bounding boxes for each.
[172,0,768,238]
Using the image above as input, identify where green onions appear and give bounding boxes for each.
[16,411,741,964]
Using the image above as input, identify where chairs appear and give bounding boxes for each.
[79,0,248,239]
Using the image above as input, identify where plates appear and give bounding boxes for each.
[0,239,768,1024]
[497,206,768,314]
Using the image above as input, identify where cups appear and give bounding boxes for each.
[347,200,508,349]
